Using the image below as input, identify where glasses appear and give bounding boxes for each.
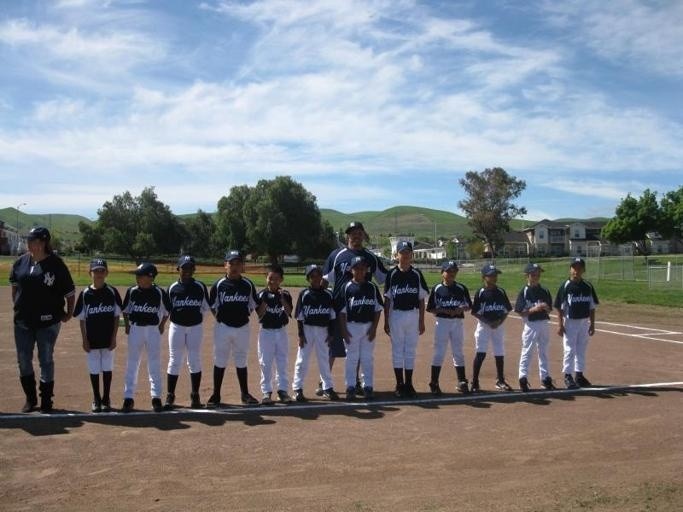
[93,269,106,273]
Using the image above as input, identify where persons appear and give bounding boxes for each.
[471,265,513,394]
[315,221,388,398]
[291,264,343,403]
[7,227,76,415]
[425,260,472,395]
[121,262,172,412]
[72,259,122,414]
[255,265,293,406]
[554,257,599,391]
[165,256,210,409]
[382,240,430,398]
[514,262,557,393]
[206,250,259,406]
[333,256,385,402]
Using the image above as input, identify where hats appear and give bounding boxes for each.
[225,249,242,261]
[21,226,52,241]
[128,262,158,277]
[177,254,196,268]
[441,260,459,273]
[305,263,323,278]
[397,241,412,253]
[89,258,108,270]
[480,264,501,276]
[523,263,541,273]
[344,221,364,234]
[349,255,370,270]
[570,257,585,268]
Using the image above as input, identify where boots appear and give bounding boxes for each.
[38,380,55,414]
[19,371,37,413]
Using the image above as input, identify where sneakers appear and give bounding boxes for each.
[151,398,162,412]
[355,382,364,395]
[292,389,306,402]
[315,383,323,396]
[277,390,292,404]
[428,382,441,393]
[323,388,340,401]
[519,377,531,392]
[261,392,273,405]
[404,383,417,398]
[92,400,100,412]
[346,385,356,401]
[122,398,135,412]
[241,394,259,404]
[455,378,469,393]
[539,377,557,391]
[207,394,221,408]
[394,383,404,398]
[494,377,513,392]
[564,376,577,388]
[164,392,175,410]
[363,386,375,398]
[575,376,592,387]
[470,378,479,392]
[190,392,201,408]
[100,398,111,412]
